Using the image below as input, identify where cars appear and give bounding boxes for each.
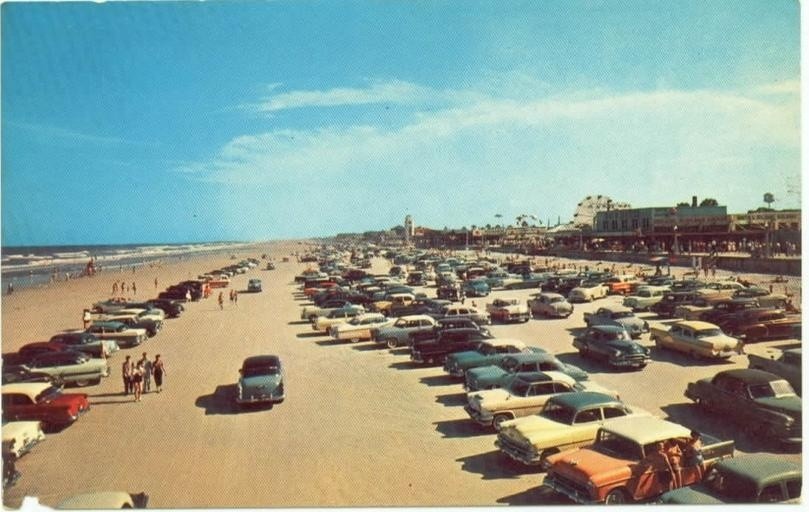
[543,419,735,504]
[235,353,283,409]
[468,354,590,395]
[462,372,618,432]
[296,242,529,344]
[441,338,551,380]
[410,327,497,366]
[574,323,651,370]
[649,319,741,363]
[509,257,802,346]
[145,256,291,320]
[683,369,802,441]
[653,452,800,504]
[2,298,185,510]
[496,394,656,465]
[742,340,802,379]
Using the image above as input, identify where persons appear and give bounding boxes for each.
[664,438,683,489]
[655,441,676,493]
[684,429,707,485]
[290,236,801,314]
[8,252,238,357]
[122,351,165,402]
